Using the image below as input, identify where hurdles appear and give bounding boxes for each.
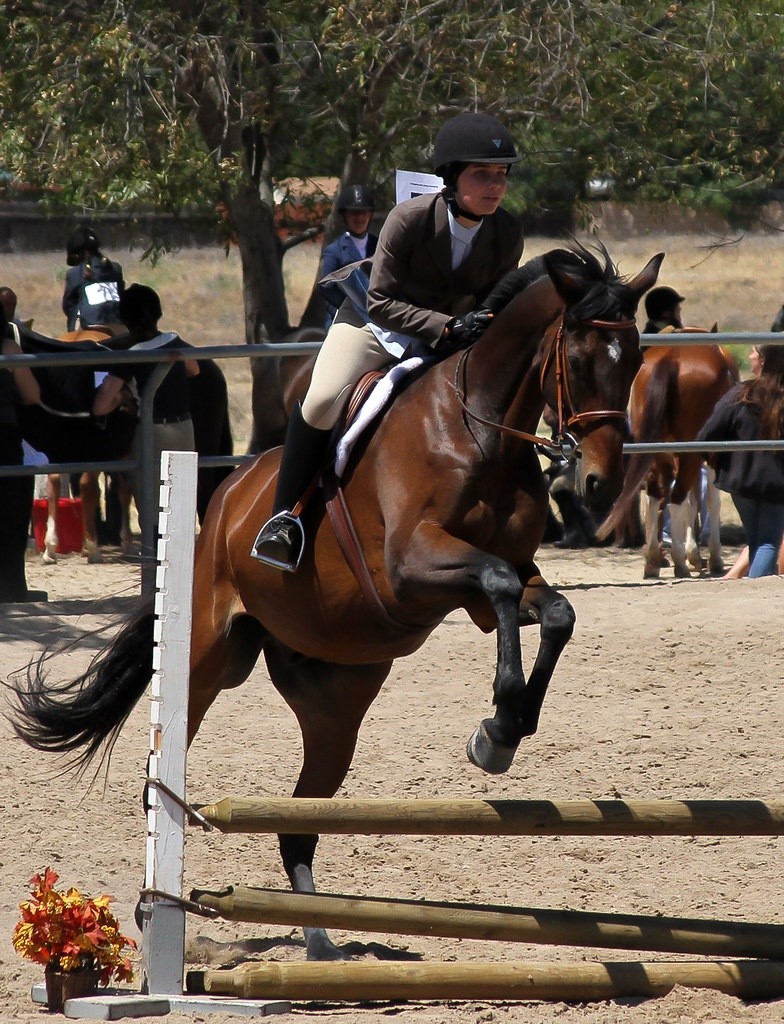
[135,449,784,999]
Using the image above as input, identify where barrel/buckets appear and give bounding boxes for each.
[33,497,83,553]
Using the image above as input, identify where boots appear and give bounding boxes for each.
[255,397,341,574]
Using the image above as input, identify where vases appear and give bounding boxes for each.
[44,962,96,1013]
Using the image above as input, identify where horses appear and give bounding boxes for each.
[6,312,237,603]
[590,328,727,578]
[1,244,666,967]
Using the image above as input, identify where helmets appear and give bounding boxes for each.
[337,185,375,215]
[66,226,95,266]
[431,113,523,177]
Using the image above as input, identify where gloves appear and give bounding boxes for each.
[443,309,494,348]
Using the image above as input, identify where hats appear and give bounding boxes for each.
[113,282,163,323]
[644,286,686,319]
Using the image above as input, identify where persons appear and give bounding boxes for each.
[299,185,379,332]
[93,283,198,539]
[694,343,784,579]
[252,113,524,571]
[534,409,589,550]
[641,287,720,552]
[0,286,47,603]
[62,227,126,331]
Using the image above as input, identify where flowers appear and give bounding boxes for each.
[12,867,138,987]
